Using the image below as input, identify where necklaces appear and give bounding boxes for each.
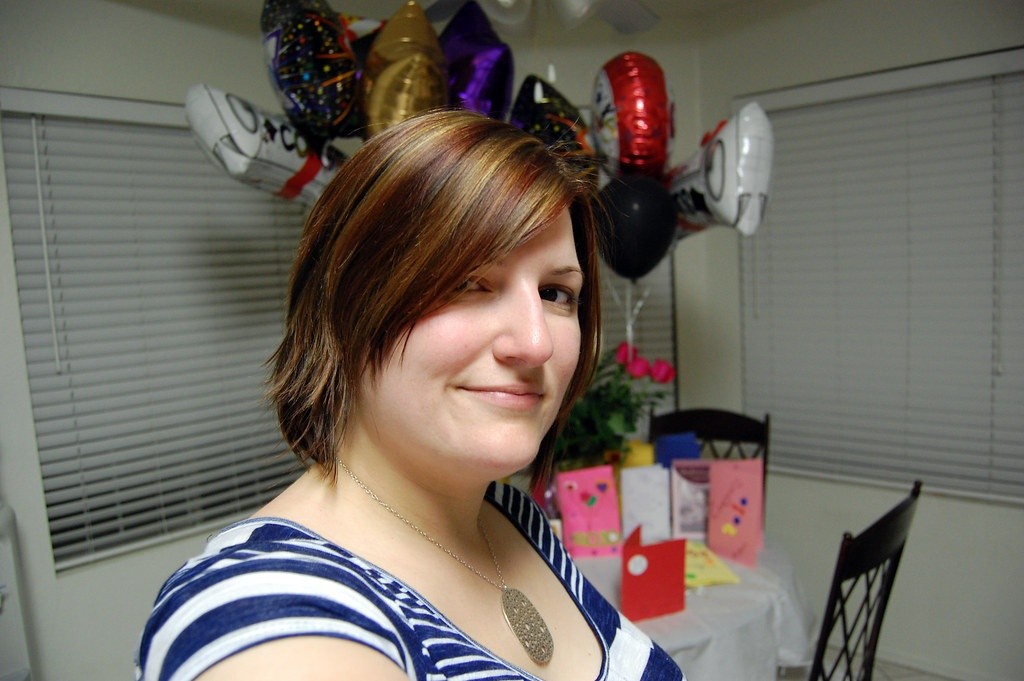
[332,452,555,670]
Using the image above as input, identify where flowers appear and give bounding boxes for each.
[517,341,678,478]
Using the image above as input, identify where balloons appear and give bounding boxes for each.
[185,0,772,281]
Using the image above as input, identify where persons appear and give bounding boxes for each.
[136,109,688,681]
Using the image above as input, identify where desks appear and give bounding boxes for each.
[573,538,817,681]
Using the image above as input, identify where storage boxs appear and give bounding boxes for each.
[669,458,708,540]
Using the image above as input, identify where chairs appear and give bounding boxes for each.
[807,477,924,681]
[648,406,770,495]
